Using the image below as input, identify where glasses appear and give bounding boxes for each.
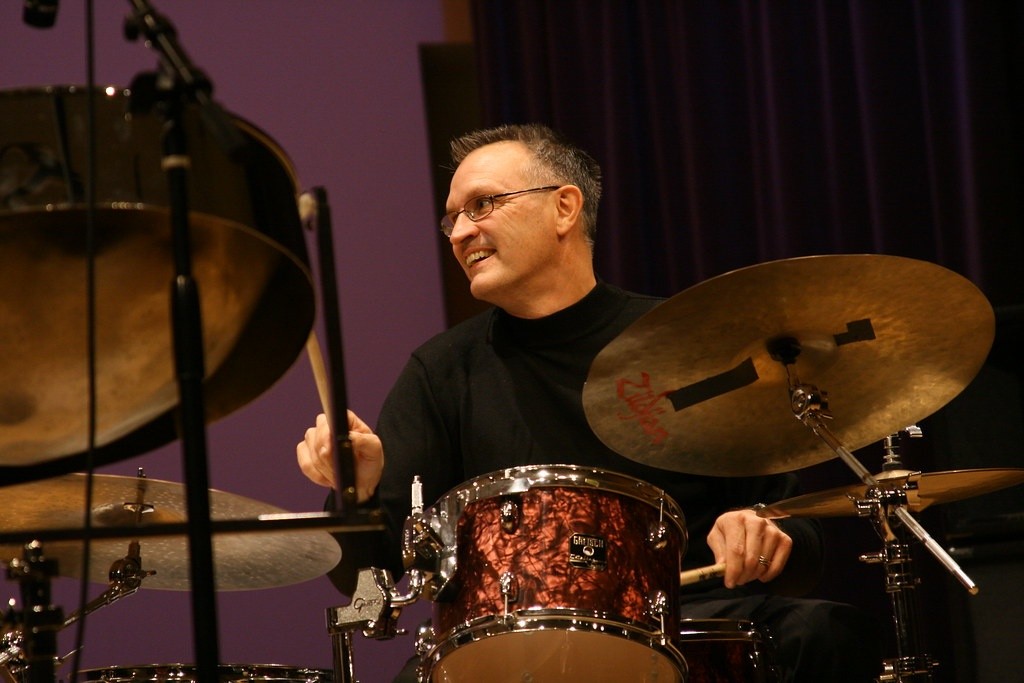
[441,186,560,238]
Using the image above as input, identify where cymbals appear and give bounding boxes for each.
[578,251,999,480]
[1,469,345,594]
[756,465,1024,520]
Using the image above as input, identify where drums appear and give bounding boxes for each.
[71,662,338,683]
[1,78,320,490]
[680,616,790,683]
[421,462,691,682]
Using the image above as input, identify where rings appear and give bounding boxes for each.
[759,556,771,567]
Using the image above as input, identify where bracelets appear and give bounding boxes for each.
[754,503,767,508]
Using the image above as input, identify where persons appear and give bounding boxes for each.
[297,125,857,683]
